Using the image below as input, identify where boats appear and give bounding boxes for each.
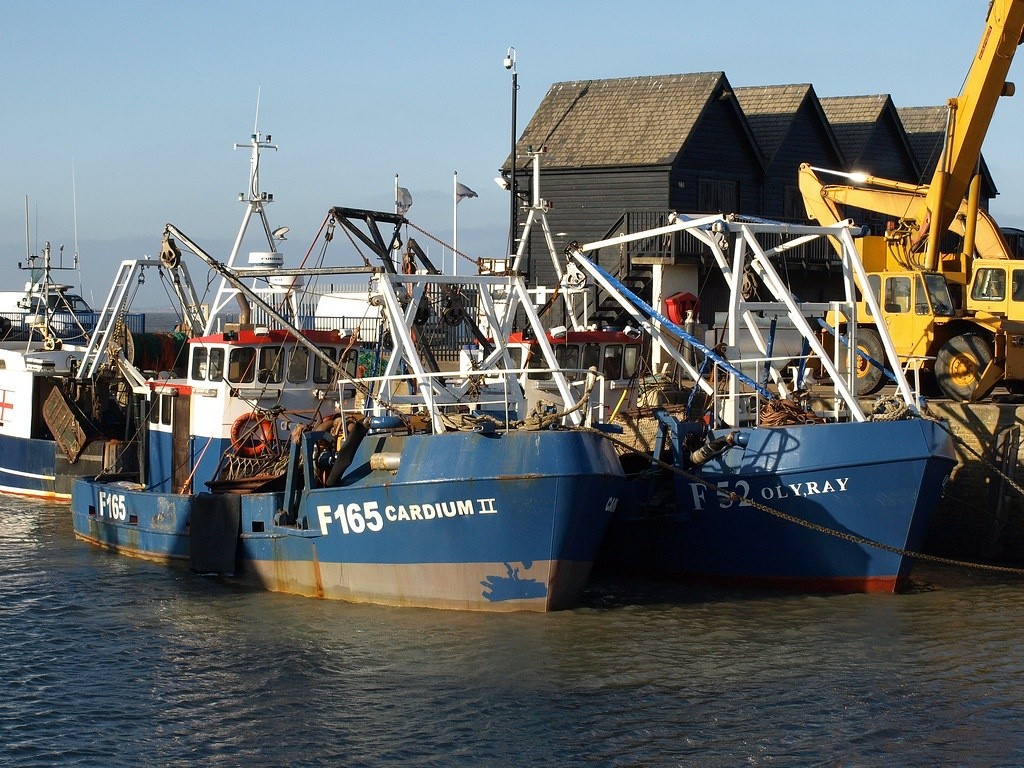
[0,82,959,614]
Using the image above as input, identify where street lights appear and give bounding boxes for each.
[502,46,519,270]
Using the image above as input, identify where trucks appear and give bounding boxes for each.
[1,291,97,338]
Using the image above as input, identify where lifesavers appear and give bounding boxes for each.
[230,412,275,455]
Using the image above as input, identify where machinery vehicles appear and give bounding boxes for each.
[797,0,1024,402]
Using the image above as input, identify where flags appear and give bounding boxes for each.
[456,182,478,204]
[397,187,413,216]
